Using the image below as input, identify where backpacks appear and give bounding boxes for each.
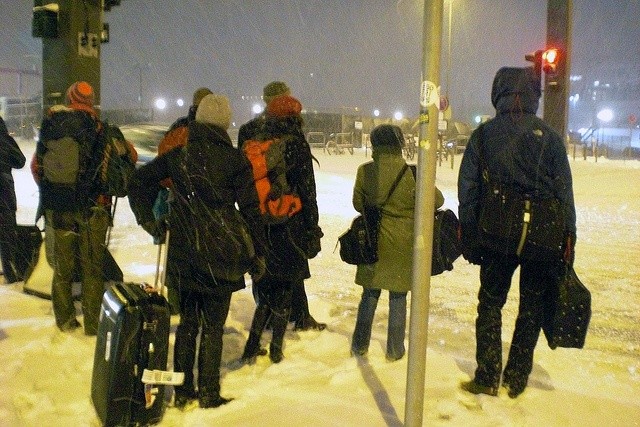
[240,132,303,226]
[94,118,136,197]
[39,116,99,211]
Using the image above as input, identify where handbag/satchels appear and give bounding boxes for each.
[541,258,591,350]
[478,181,566,261]
[186,209,255,282]
[431,208,463,276]
[338,210,382,265]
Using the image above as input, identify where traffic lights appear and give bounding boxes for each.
[525,50,542,82]
[542,46,564,76]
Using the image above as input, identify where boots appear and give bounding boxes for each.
[242,303,272,358]
[270,305,291,363]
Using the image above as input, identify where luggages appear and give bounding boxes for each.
[91,231,170,427]
[15,226,42,279]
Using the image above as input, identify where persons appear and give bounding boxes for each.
[127,94,266,408]
[241,92,321,361]
[458,66,577,396]
[0,114,27,283]
[350,124,444,359]
[237,82,328,330]
[156,86,212,317]
[30,81,134,335]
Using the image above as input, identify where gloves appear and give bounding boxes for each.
[307,225,323,259]
[461,240,484,265]
[248,256,267,283]
[141,218,167,237]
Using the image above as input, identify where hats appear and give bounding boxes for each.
[195,94,231,131]
[266,95,302,117]
[369,124,405,147]
[263,80,291,103]
[193,87,214,105]
[66,81,95,107]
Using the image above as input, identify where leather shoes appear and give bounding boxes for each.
[173,392,198,410]
[199,390,233,409]
[292,315,327,331]
[501,376,523,399]
[461,381,498,398]
